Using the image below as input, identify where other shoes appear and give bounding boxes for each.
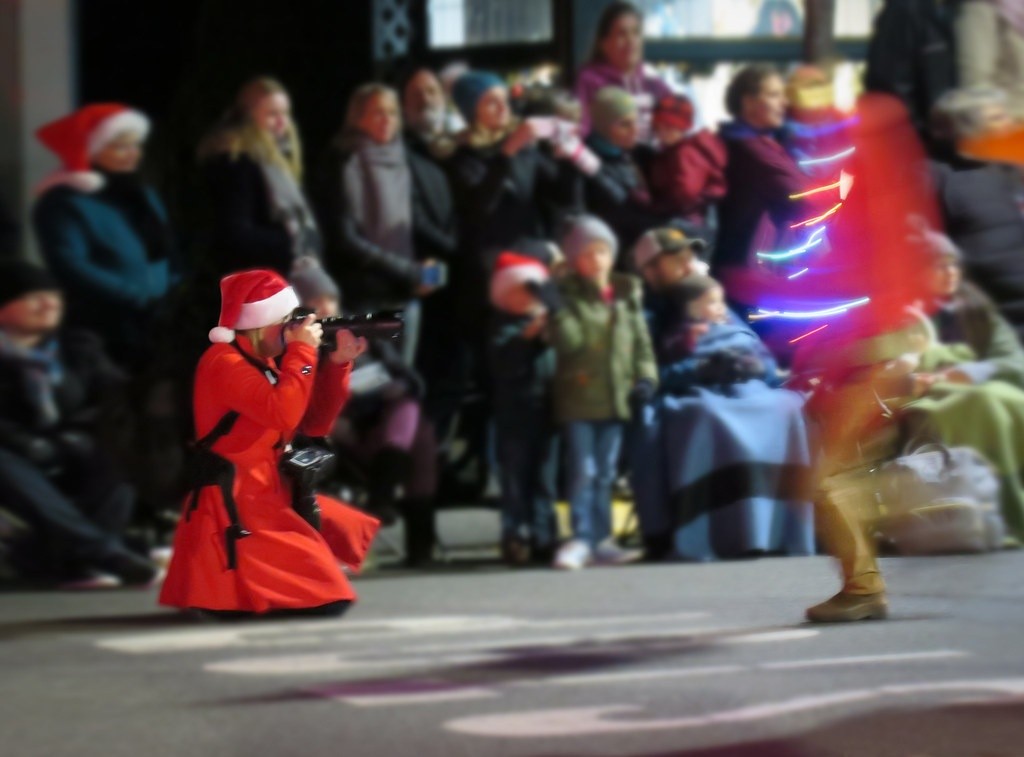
[503,537,533,564]
[553,537,592,571]
[806,591,887,625]
[600,540,643,563]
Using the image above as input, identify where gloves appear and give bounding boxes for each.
[524,277,563,311]
[715,351,765,380]
[628,379,654,406]
[700,354,741,383]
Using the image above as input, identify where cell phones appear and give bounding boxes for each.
[419,262,448,287]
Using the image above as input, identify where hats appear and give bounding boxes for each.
[895,213,957,265]
[678,273,715,300]
[650,93,696,134]
[626,229,710,276]
[489,250,550,310]
[209,271,299,345]
[287,266,337,298]
[554,212,617,260]
[1,259,59,314]
[589,84,636,134]
[450,70,505,118]
[35,102,151,170]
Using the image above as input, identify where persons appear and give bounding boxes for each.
[861,0,1024,182]
[198,67,446,570]
[889,220,1024,555]
[449,1,832,573]
[777,60,940,623]
[154,265,380,619]
[0,257,171,593]
[923,80,1024,339]
[733,0,805,75]
[27,98,196,536]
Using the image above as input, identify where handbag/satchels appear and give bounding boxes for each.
[876,442,1004,555]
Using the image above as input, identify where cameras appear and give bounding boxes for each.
[281,305,404,356]
